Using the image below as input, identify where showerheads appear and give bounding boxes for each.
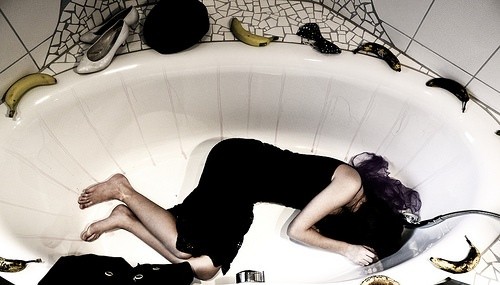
[402,212,444,230]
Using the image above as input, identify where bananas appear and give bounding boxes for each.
[0,256,44,273]
[428,236,481,274]
[3,72,57,117]
[427,77,471,112]
[359,274,400,285]
[354,42,402,71]
[231,17,279,47]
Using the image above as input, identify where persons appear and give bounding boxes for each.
[75,138,426,279]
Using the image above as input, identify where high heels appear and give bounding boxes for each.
[79,5,139,43]
[74,20,128,75]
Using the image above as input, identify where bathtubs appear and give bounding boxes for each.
[0,42,500,285]
